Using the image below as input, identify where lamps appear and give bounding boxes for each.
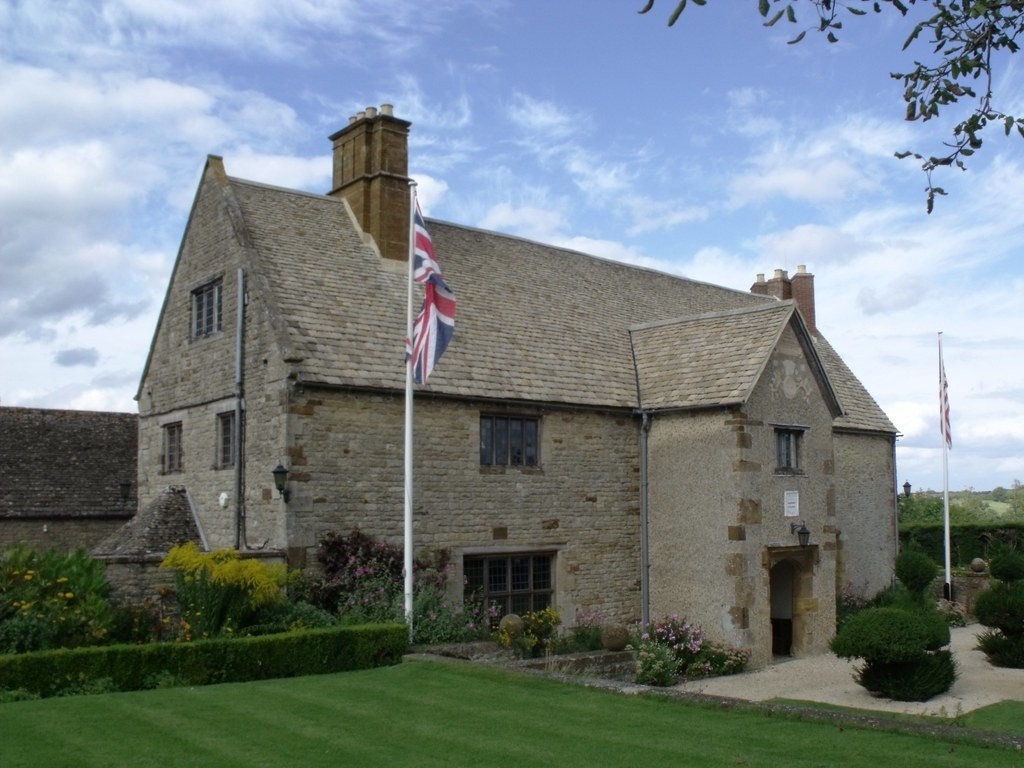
[271,459,291,503]
[790,520,810,551]
[897,479,912,503]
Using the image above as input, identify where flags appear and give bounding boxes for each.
[404,195,456,384]
[940,349,952,450]
